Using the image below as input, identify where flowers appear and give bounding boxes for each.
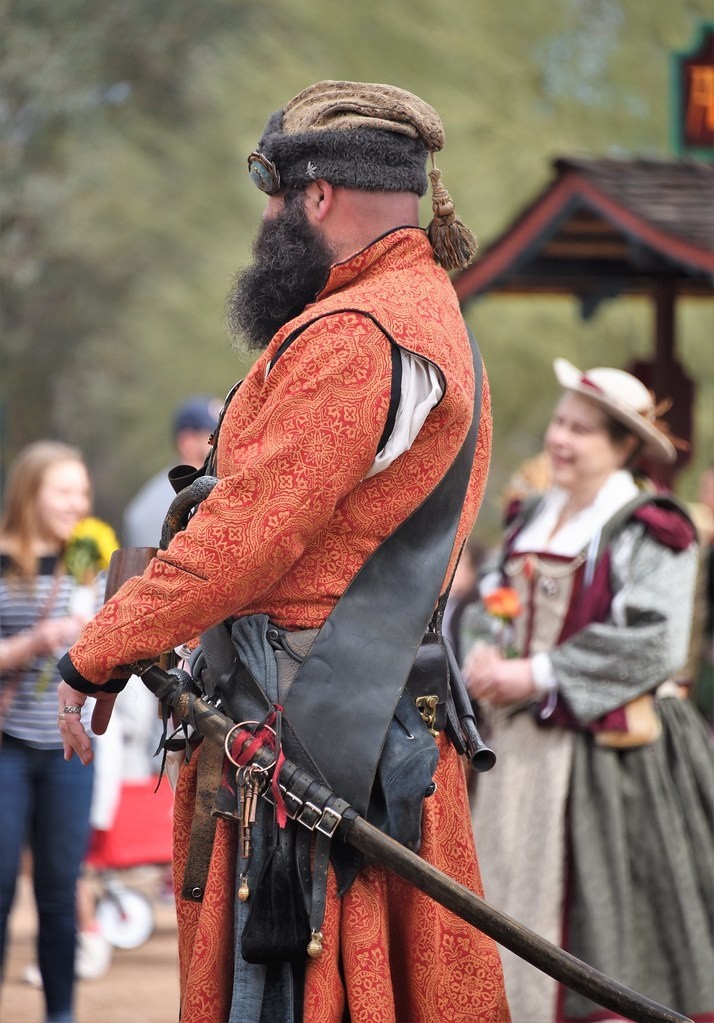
[467,584,525,662]
[64,517,122,582]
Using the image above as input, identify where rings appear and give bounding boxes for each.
[58,714,66,721]
[64,705,82,713]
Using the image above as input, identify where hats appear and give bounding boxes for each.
[248,79,479,273]
[553,357,688,464]
[170,396,225,432]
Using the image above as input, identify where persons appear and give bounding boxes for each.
[435,350,714,1022]
[18,349,703,986]
[57,77,526,1022]
[0,440,109,1023]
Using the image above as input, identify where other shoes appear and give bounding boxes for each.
[23,965,43,988]
[74,931,112,978]
